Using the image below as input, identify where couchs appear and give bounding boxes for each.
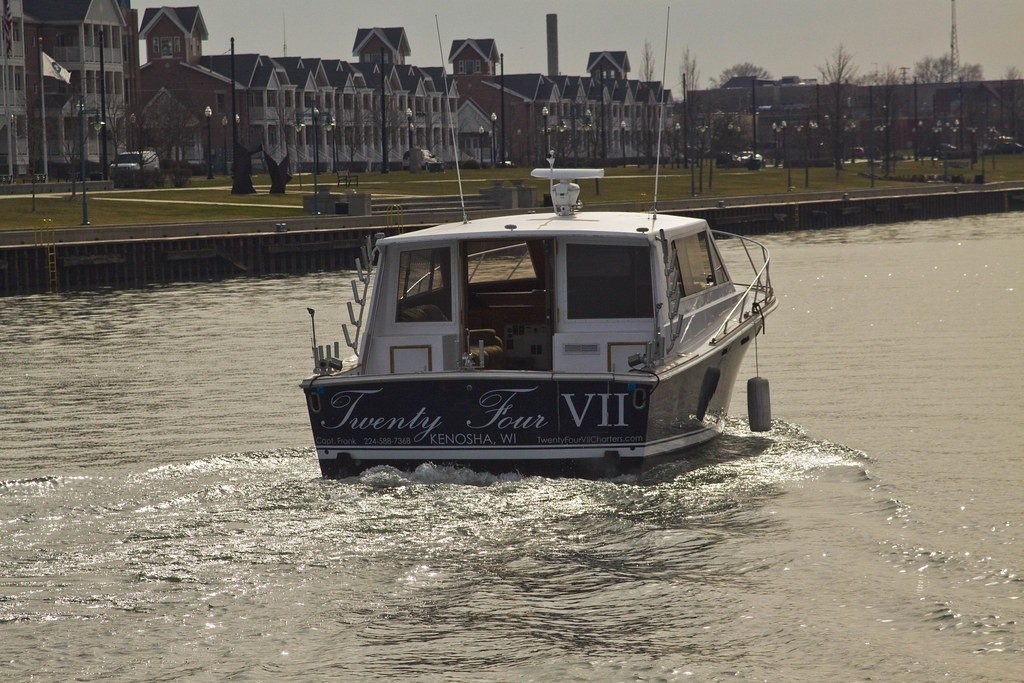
[400,304,504,368]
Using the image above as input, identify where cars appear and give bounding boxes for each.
[732,150,762,166]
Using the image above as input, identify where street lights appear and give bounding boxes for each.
[326,116,337,174]
[583,109,591,160]
[292,123,305,163]
[490,112,497,164]
[313,107,321,176]
[76,103,84,181]
[488,130,494,164]
[621,121,627,168]
[409,124,415,151]
[129,111,136,153]
[541,106,551,164]
[479,125,485,163]
[675,123,681,167]
[221,116,229,175]
[205,105,214,180]
[406,106,413,148]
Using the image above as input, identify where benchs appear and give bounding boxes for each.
[879,157,896,172]
[335,170,358,188]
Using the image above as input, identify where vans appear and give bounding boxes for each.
[111,151,160,177]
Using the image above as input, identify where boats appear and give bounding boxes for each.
[297,162,781,475]
